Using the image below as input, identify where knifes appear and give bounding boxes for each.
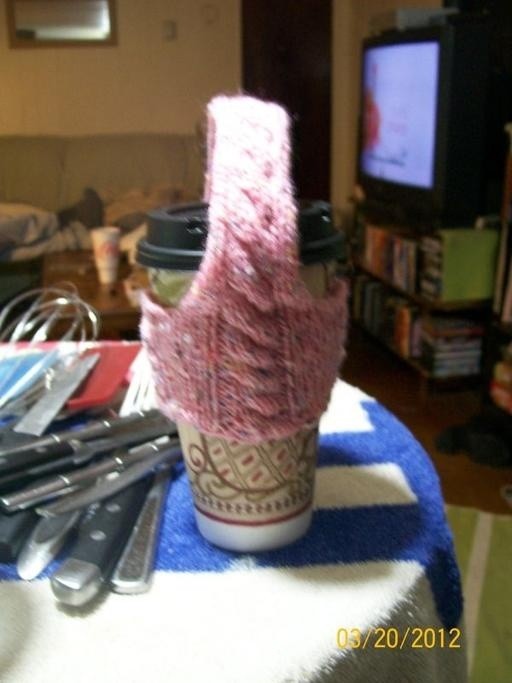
[0,351,184,607]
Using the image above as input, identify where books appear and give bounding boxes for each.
[351,224,497,378]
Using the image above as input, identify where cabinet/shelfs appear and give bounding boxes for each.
[346,189,501,403]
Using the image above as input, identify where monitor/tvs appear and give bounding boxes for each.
[355,28,496,222]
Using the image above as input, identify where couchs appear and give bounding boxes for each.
[2,132,205,317]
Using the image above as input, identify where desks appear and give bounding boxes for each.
[40,249,146,340]
[2,338,447,683]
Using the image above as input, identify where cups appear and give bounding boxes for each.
[91,226,121,286]
[131,197,348,552]
